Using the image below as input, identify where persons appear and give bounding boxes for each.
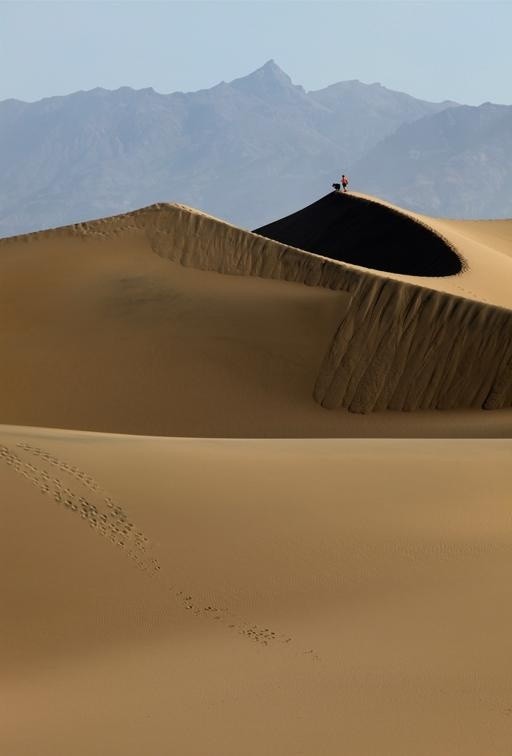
[332,175,348,192]
